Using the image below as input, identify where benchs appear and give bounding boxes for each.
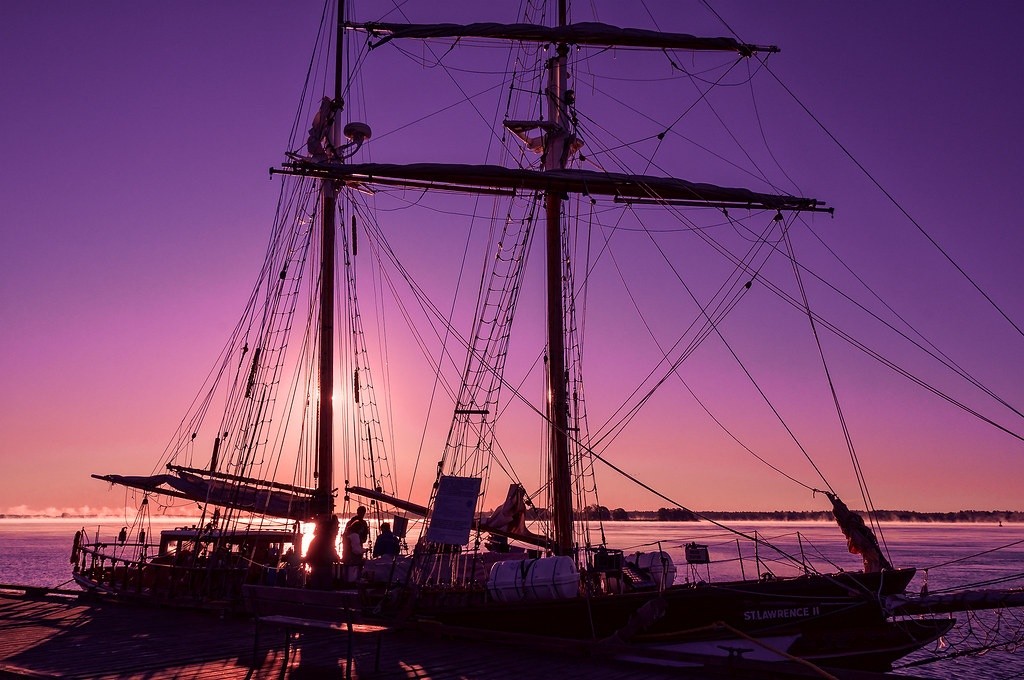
[242,586,393,680]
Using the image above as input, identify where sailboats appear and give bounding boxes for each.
[77,0,956,680]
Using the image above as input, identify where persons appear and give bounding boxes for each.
[341,505,401,561]
